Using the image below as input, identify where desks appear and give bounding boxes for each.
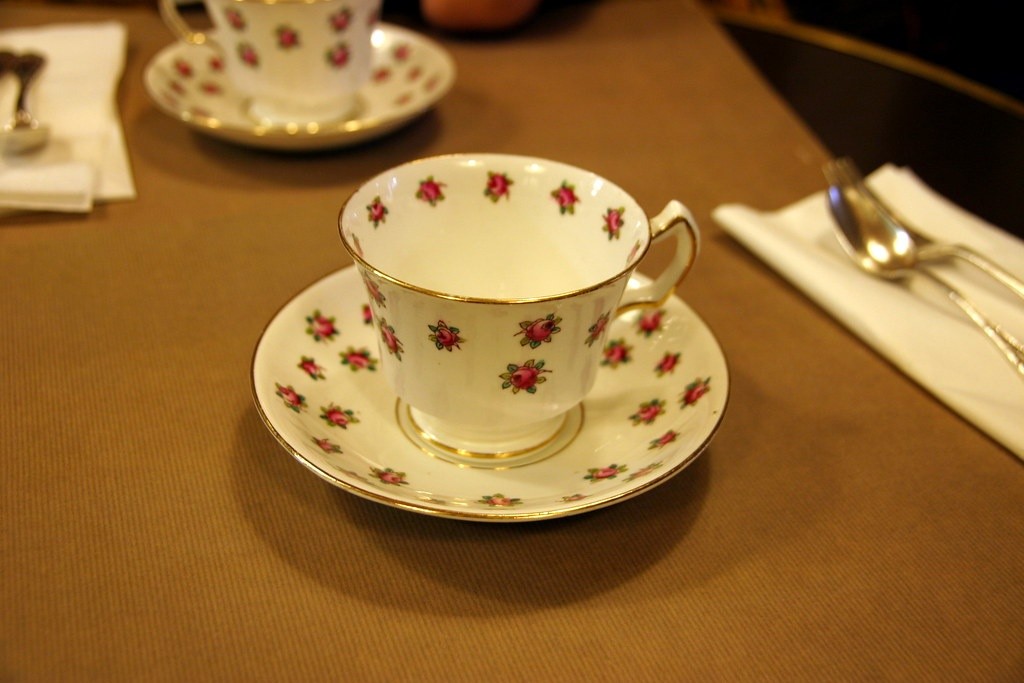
[0,0,1023,683]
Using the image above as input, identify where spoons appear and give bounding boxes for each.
[825,184,1023,380]
[0,52,49,153]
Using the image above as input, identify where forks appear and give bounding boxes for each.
[820,156,1023,301]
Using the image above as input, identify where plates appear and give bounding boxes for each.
[142,22,456,154]
[249,264,730,523]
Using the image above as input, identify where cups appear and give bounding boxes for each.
[336,152,701,460]
[156,0,384,124]
